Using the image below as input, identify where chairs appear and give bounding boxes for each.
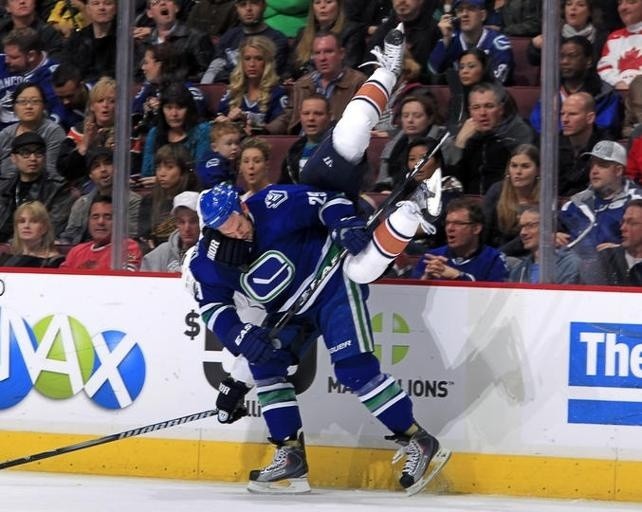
[0,14,578,283]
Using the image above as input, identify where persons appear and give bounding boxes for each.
[180,28,467,425]
[0,0,641,290]
[187,179,440,489]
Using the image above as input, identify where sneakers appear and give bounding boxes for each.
[382,30,403,76]
[384,429,439,486]
[250,437,308,480]
[416,180,445,223]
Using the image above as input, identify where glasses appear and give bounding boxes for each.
[517,221,538,229]
[16,148,44,158]
[15,96,42,106]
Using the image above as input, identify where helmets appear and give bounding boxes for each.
[180,244,204,302]
[199,182,239,227]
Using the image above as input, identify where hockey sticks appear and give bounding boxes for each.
[270,69,461,340]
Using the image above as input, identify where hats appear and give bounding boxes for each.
[86,147,113,168]
[13,131,45,148]
[170,191,199,216]
[580,140,627,165]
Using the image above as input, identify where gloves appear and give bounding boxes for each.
[216,380,249,423]
[233,323,279,367]
[329,215,370,255]
